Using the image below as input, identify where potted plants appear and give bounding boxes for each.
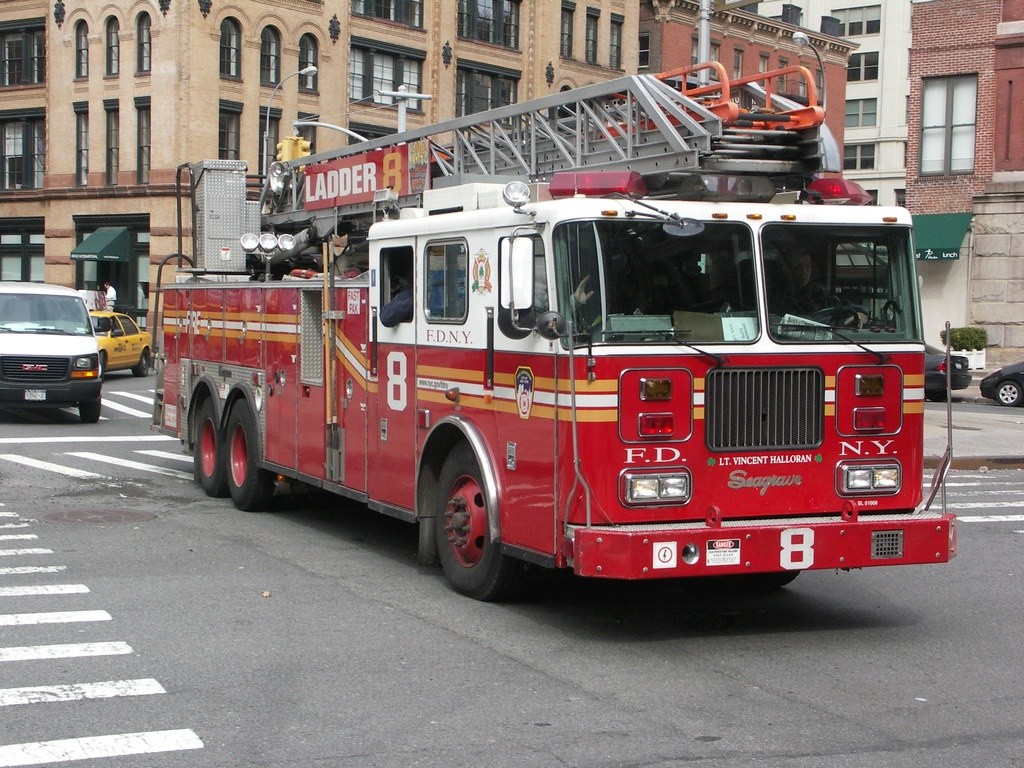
[940,326,986,372]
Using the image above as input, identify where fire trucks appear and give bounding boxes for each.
[151,60,958,603]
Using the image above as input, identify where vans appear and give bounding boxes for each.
[0,281,103,423]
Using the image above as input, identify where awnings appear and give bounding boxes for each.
[836,212,973,261]
[70,227,129,262]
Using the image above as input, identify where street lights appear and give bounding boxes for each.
[262,66,318,184]
[793,32,826,122]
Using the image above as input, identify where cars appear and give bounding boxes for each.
[980,362,1024,407]
[925,344,972,403]
[88,311,152,381]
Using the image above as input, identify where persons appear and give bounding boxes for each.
[770,246,869,326]
[104,281,117,332]
[534,274,645,333]
[379,278,412,327]
